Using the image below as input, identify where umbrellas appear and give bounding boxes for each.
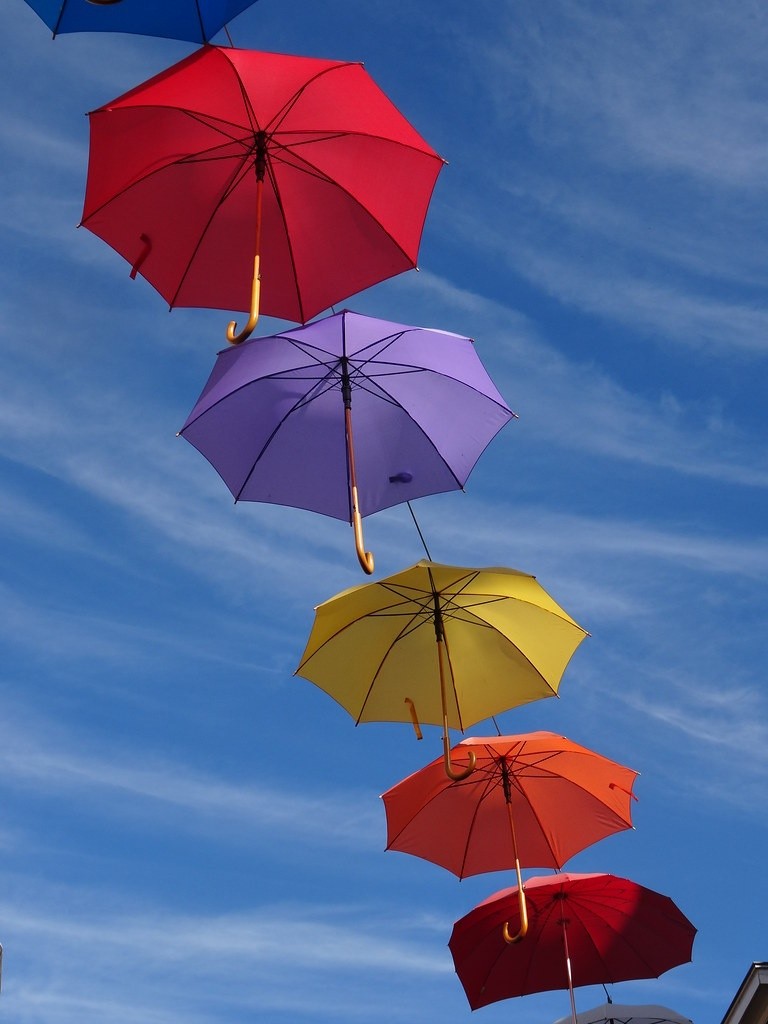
[450,872,698,1024]
[27,0,259,49]
[77,44,448,343]
[379,731,643,943]
[293,559,593,780]
[554,1003,695,1024]
[178,308,516,575]
